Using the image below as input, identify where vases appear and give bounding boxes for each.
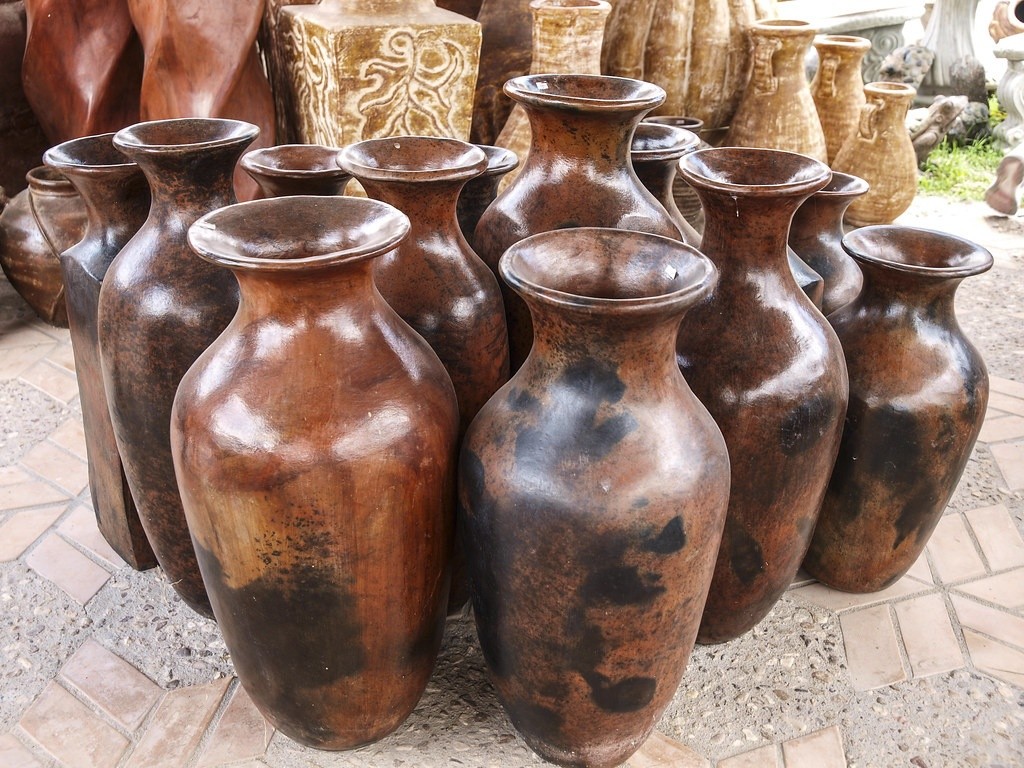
[0,0,997,768]
